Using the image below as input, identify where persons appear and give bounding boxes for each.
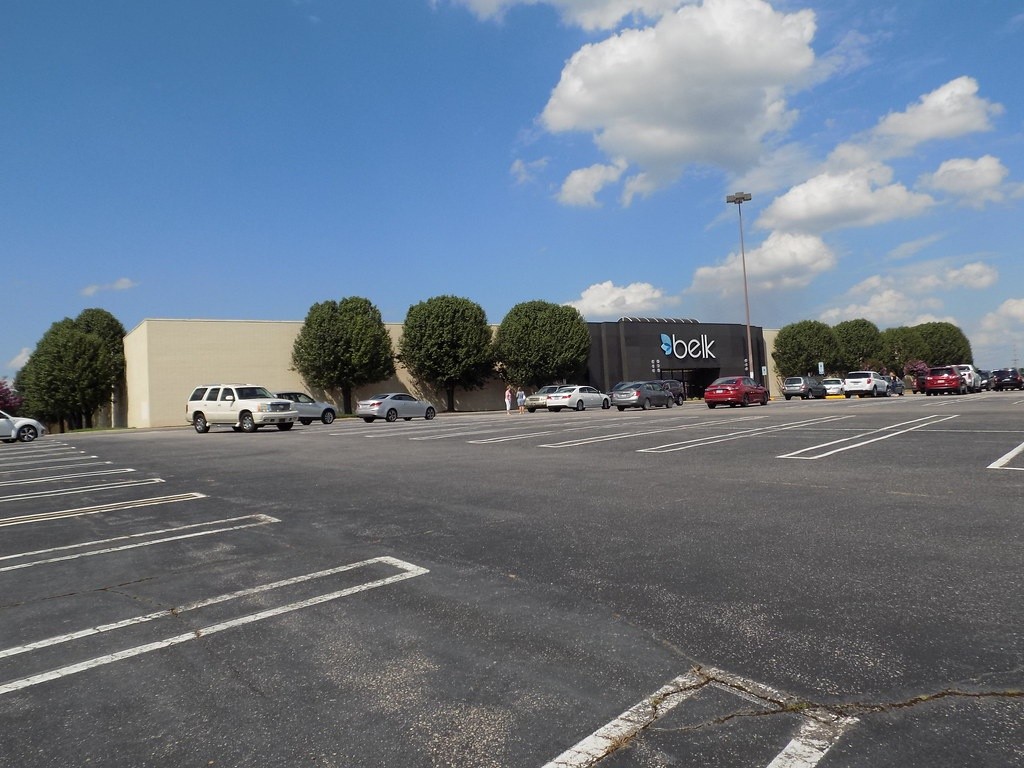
[504,385,513,415]
[516,387,526,414]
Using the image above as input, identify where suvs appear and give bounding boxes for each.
[525,384,576,413]
[186,384,298,433]
[654,379,684,406]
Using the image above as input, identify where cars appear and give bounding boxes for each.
[974,369,995,392]
[547,386,611,412]
[606,381,639,406]
[946,364,981,394]
[916,366,967,395]
[880,376,905,396]
[846,371,891,398]
[705,376,771,408]
[821,378,845,396]
[271,390,337,424]
[0,410,46,444]
[912,377,924,394]
[995,367,1023,392]
[782,376,827,400]
[990,370,1001,391]
[355,393,436,422]
[613,381,674,411]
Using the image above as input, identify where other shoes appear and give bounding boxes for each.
[519,412,525,415]
[507,413,512,415]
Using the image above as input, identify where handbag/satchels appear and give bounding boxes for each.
[522,391,526,401]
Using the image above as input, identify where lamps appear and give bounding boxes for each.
[727,192,751,204]
